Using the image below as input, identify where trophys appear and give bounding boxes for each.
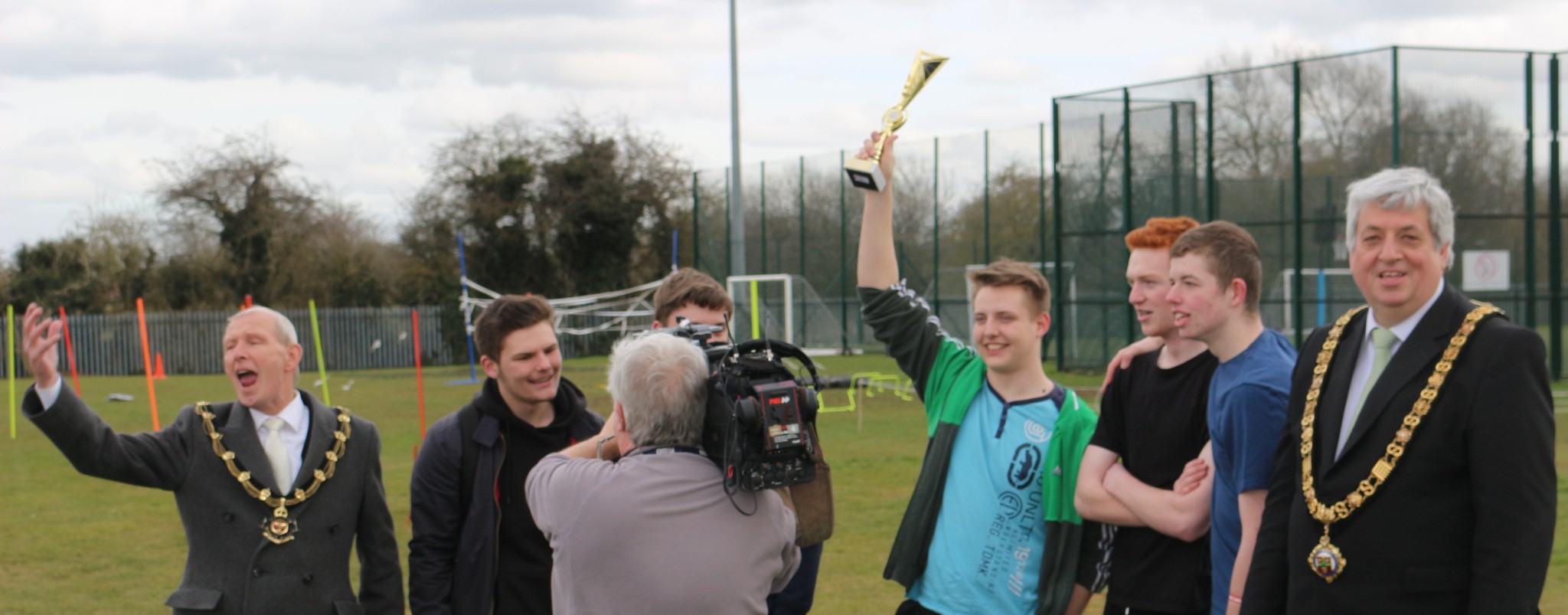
[844,51,951,192]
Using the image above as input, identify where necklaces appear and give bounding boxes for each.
[196,400,351,545]
[1299,302,1506,585]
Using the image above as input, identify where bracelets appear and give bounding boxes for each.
[1228,595,1242,604]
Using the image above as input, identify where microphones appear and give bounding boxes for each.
[636,324,723,337]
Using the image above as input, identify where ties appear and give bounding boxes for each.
[262,419,292,496]
[1346,328,1398,448]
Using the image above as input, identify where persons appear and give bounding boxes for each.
[856,131,1100,615]
[407,293,608,615]
[1073,216,1218,615]
[1103,220,1297,615]
[23,302,406,615]
[653,266,821,615]
[523,333,801,615]
[1235,165,1558,615]
